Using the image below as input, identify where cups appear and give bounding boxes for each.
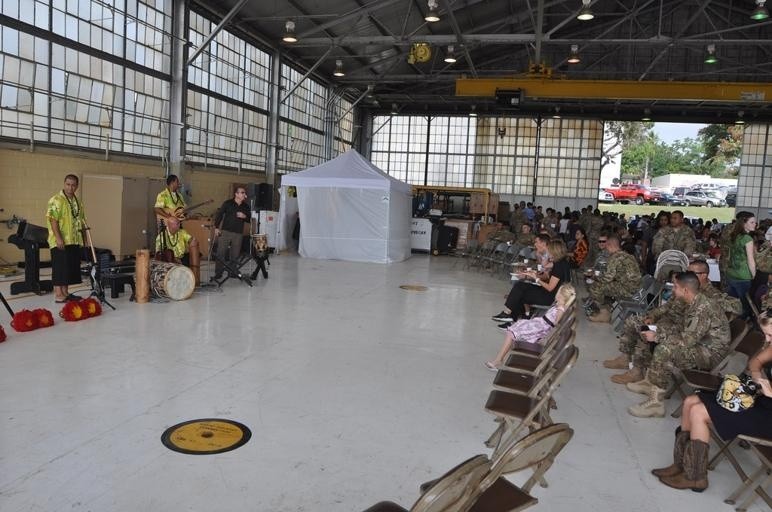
[694,270,707,275]
[237,191,246,194]
[598,240,606,243]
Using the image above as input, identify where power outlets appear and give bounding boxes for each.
[604,184,660,205]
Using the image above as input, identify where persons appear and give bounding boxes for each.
[652,290,772,492]
[155,217,199,267]
[154,175,189,235]
[472,202,772,331]
[45,175,84,303]
[215,186,251,279]
[604,260,743,384]
[627,270,732,417]
[484,283,577,372]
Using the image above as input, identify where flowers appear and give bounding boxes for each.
[201,224,212,228]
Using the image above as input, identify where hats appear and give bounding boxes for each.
[654,193,685,206]
[672,187,691,196]
[726,194,736,207]
[598,189,614,203]
[691,183,737,191]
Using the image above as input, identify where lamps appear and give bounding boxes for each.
[420,423,573,511]
[510,304,576,354]
[360,455,493,512]
[491,330,579,396]
[669,327,766,418]
[501,315,578,374]
[664,312,746,400]
[482,344,579,447]
[452,230,579,285]
[708,423,772,484]
[609,273,664,330]
[738,445,771,512]
[732,290,765,350]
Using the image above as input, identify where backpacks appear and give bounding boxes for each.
[254,233,267,250]
[135,259,195,301]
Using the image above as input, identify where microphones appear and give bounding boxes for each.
[103,273,136,298]
[246,249,275,279]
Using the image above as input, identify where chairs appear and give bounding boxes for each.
[216,274,222,279]
[229,273,241,277]
[496,323,515,332]
[491,311,514,323]
[483,359,500,371]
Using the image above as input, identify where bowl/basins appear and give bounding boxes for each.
[168,188,180,205]
[166,227,178,246]
[63,189,80,218]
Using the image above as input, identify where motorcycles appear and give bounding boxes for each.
[82,229,117,310]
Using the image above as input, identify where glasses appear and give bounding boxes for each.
[365,85,377,99]
[468,105,478,117]
[576,0,595,22]
[283,20,298,42]
[705,45,719,65]
[424,0,440,22]
[389,102,398,116]
[333,59,345,78]
[567,45,582,64]
[750,0,770,20]
[443,44,457,63]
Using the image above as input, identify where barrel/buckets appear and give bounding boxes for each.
[69,294,82,299]
[54,297,72,304]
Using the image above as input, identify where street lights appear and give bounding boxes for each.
[714,371,756,414]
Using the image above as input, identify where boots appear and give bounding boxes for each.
[683,191,726,208]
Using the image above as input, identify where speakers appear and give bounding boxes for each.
[610,369,645,383]
[650,426,691,478]
[658,439,710,493]
[626,384,665,418]
[587,307,610,322]
[626,379,653,395]
[601,352,632,370]
